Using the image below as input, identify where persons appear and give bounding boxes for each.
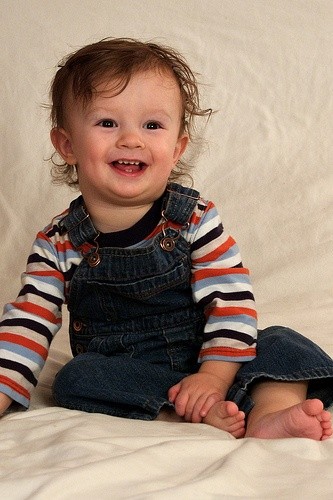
[0,36,333,441]
